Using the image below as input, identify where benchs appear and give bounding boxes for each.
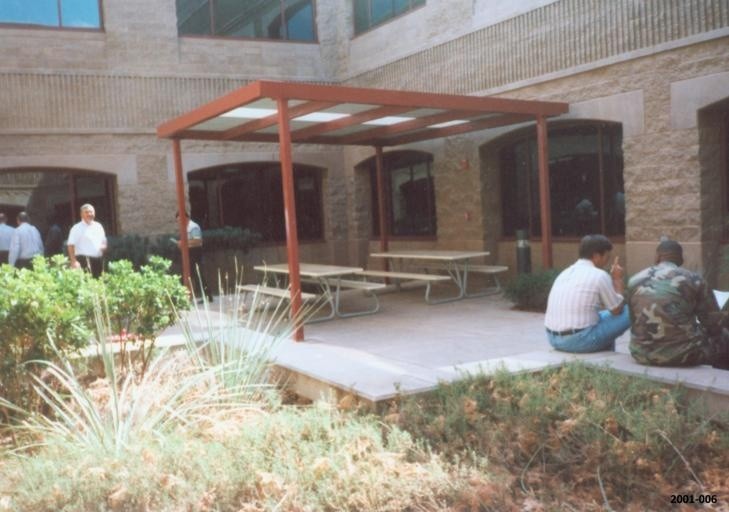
[236,263,509,326]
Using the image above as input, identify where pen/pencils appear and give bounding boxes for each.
[611,256,619,274]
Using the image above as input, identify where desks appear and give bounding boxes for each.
[364,251,502,303]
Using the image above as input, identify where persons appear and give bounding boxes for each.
[41,215,64,254]
[541,232,632,356]
[67,203,108,281]
[8,210,45,271]
[573,190,597,237]
[606,183,625,234]
[625,239,728,368]
[0,212,16,266]
[171,210,214,304]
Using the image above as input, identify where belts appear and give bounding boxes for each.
[546,328,584,336]
